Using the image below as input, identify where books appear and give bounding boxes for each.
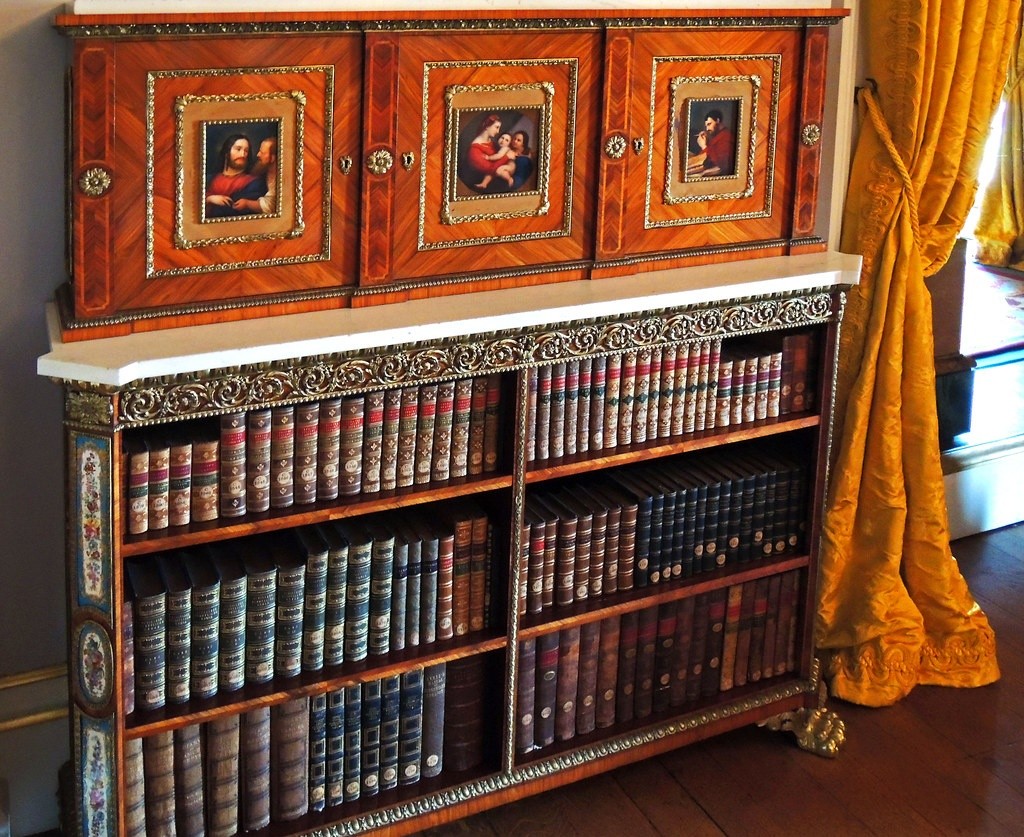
[121,330,812,837]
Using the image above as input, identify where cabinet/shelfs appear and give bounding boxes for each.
[38,283,853,837]
